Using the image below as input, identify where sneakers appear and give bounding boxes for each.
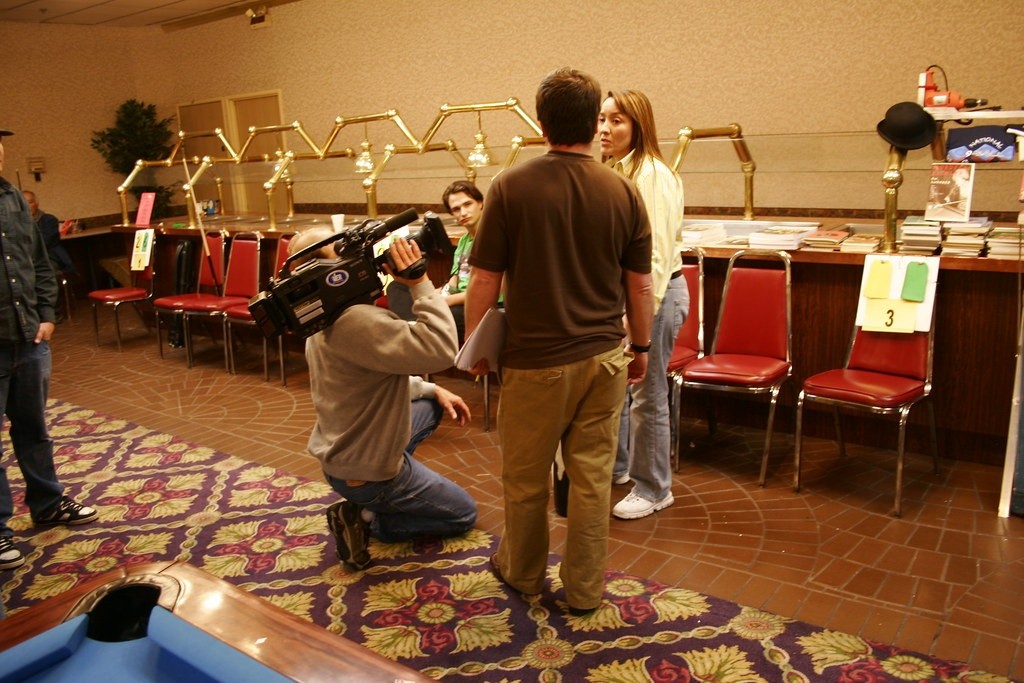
[32,496,99,525]
[613,489,677,520]
[615,472,631,485]
[326,502,372,570]
[1,537,26,569]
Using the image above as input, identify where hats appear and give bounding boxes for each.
[877,101,937,151]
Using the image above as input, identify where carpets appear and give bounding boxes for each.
[0,398,1013,683]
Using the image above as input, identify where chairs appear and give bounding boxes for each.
[89,231,305,386]
[793,301,943,516]
[666,245,714,467]
[674,249,800,486]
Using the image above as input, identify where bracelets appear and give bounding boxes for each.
[630,340,652,352]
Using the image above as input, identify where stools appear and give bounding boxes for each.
[423,342,490,432]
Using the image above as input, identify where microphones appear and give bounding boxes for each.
[369,207,419,239]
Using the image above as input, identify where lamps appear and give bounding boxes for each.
[464,110,499,167]
[271,133,298,178]
[352,121,376,173]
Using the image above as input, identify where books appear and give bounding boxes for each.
[684,161,1023,261]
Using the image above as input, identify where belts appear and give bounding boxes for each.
[671,270,683,281]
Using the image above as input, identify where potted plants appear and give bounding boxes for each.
[90,97,174,220]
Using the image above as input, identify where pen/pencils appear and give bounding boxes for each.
[473,373,481,385]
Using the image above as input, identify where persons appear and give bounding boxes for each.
[465,72,654,615]
[18,189,73,324]
[599,89,690,518]
[0,128,100,569]
[288,227,478,571]
[434,181,506,328]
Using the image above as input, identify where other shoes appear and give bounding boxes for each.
[490,552,544,595]
